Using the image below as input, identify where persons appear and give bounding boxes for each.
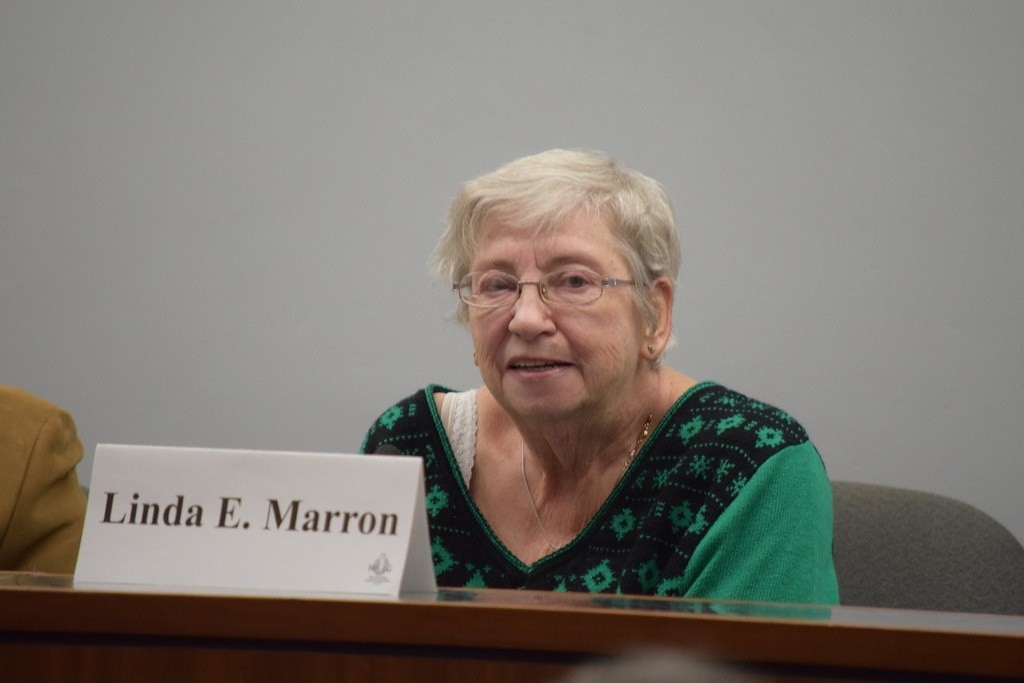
[359,149,842,606]
[1,384,87,574]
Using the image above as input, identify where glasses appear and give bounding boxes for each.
[452,268,645,308]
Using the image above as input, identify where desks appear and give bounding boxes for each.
[0,567,1024,683]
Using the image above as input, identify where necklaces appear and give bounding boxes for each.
[521,411,654,548]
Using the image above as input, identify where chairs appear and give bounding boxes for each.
[831,482,1024,615]
